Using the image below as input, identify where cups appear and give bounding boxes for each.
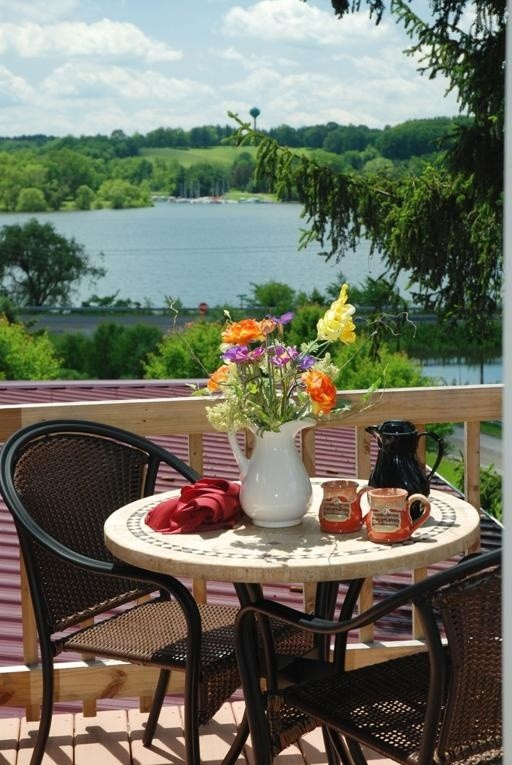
[317,478,376,534]
[362,486,431,546]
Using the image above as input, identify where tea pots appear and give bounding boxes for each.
[364,419,443,498]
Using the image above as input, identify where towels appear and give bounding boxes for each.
[141,474,239,535]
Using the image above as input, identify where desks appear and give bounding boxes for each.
[104,477,479,765]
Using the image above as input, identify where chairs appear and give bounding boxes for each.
[0,418,331,765]
[231,549,502,765]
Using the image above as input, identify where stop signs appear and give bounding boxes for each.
[199,303,208,312]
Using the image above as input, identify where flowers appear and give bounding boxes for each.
[165,282,417,439]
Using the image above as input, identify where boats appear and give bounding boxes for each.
[148,193,270,204]
